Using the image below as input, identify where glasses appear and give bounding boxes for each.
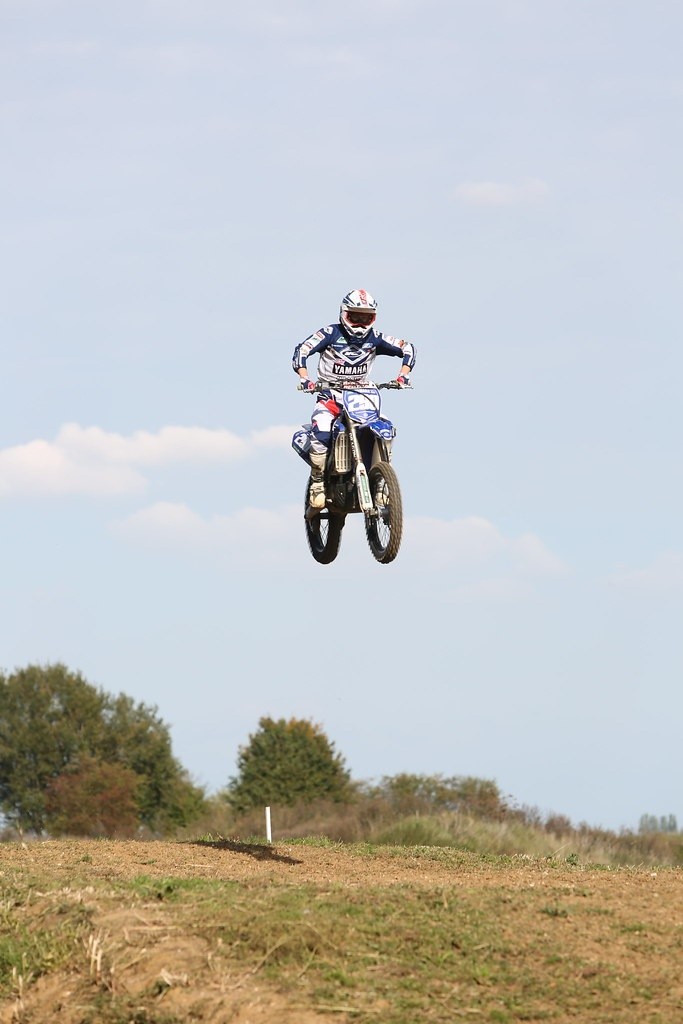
[349,315,372,324]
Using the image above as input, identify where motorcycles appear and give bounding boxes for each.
[296,380,414,565]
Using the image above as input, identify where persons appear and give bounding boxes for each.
[292,289,417,509]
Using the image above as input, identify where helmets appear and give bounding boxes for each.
[339,289,378,340]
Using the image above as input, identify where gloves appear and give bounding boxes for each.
[297,376,315,390]
[397,373,411,387]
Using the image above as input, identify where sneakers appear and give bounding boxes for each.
[309,475,326,508]
[375,482,389,506]
